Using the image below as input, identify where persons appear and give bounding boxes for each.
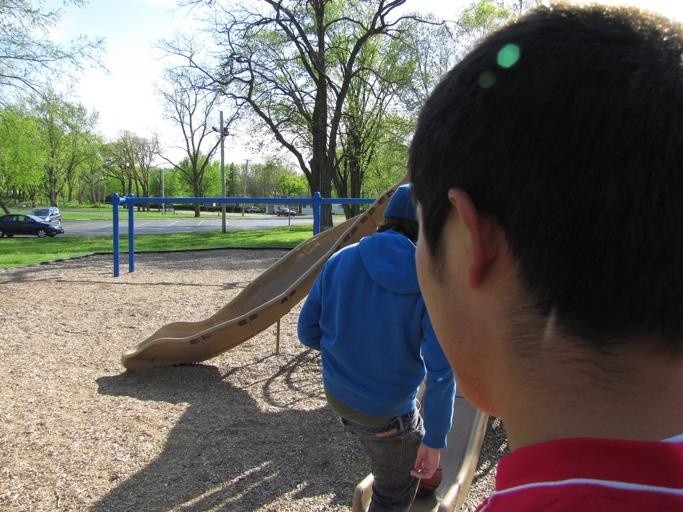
[411,4,680,512]
[295,183,455,511]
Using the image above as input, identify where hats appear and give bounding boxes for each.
[384,185,418,223]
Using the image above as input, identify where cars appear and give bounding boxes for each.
[246,206,265,213]
[0,214,64,238]
[274,207,296,216]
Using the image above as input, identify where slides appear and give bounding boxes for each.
[121,169,414,372]
[353,368,490,512]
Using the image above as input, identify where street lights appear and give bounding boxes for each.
[212,111,229,234]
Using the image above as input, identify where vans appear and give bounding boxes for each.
[30,207,62,226]
